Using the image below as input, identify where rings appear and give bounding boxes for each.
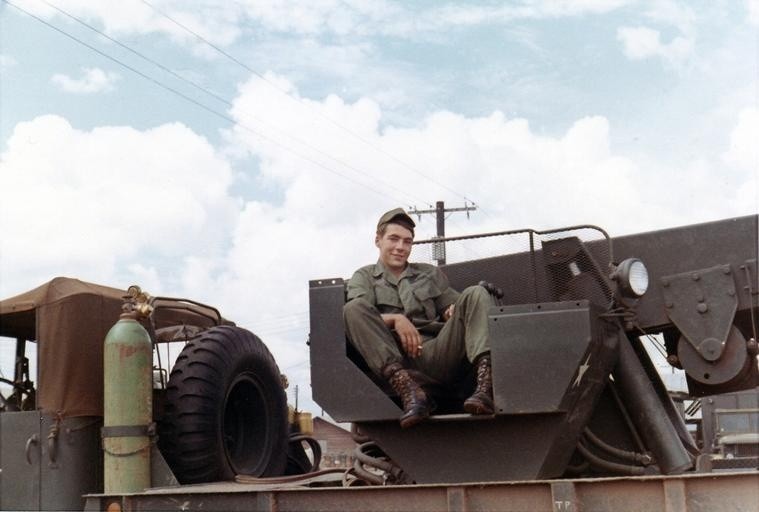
[417,344,423,349]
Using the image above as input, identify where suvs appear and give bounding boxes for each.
[0,276,290,512]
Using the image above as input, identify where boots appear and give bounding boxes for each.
[461,351,496,415]
[383,360,439,429]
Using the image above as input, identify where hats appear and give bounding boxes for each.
[376,207,417,229]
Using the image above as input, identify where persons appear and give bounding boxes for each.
[342,208,504,428]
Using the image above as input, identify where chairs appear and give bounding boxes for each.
[348,342,451,403]
